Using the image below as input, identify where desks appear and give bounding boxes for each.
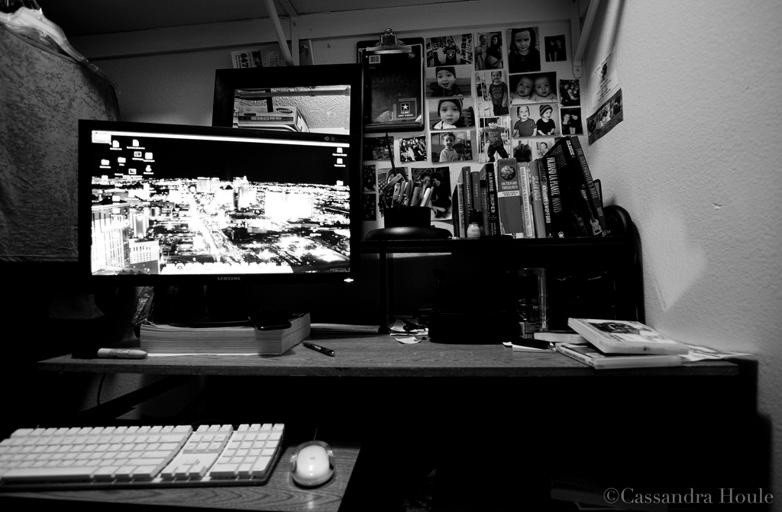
[3,321,756,511]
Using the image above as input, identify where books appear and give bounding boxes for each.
[533,315,690,370]
[452,136,606,239]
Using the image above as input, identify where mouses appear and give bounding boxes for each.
[290,441,337,490]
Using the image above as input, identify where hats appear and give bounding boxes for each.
[540,105,552,116]
[436,66,456,79]
[487,118,497,124]
[447,39,453,42]
[438,95,464,117]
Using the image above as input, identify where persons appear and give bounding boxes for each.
[439,134,460,163]
[512,74,532,104]
[488,72,508,116]
[536,105,558,136]
[532,76,557,101]
[479,119,509,162]
[510,29,539,71]
[562,110,581,133]
[511,107,537,140]
[474,33,487,68]
[564,82,580,104]
[440,37,460,61]
[487,36,503,68]
[430,66,463,97]
[433,99,466,130]
[420,174,450,216]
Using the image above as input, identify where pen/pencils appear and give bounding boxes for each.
[410,181,422,206]
[403,180,412,205]
[302,340,335,356]
[420,186,431,207]
[397,180,406,204]
[391,182,400,207]
[425,182,437,207]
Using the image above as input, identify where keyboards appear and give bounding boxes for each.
[0,422,289,494]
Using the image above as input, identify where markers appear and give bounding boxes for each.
[96,347,147,359]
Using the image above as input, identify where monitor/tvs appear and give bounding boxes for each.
[75,118,364,330]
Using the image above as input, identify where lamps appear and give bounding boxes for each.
[365,227,451,331]
[367,27,410,54]
